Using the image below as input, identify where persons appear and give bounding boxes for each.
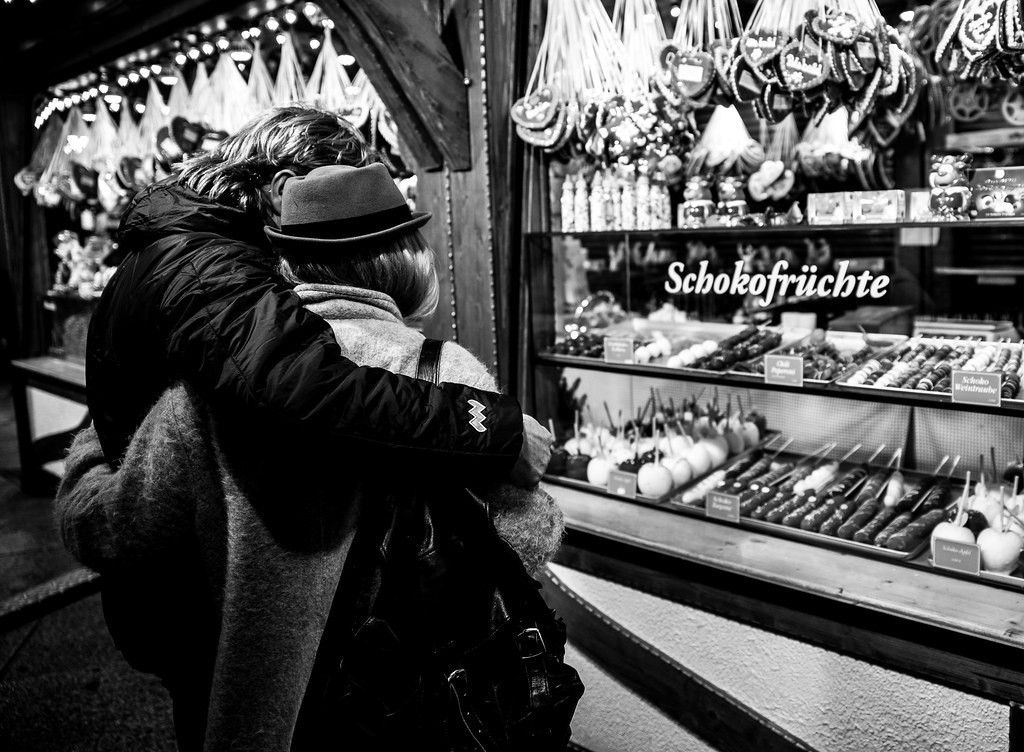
[53,163,566,752]
[86,106,555,752]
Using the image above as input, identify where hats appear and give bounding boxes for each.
[263,162,432,242]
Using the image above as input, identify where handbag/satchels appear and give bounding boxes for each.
[291,484,594,752]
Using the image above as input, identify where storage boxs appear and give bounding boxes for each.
[807,190,905,225]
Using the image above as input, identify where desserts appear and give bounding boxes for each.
[544,385,766,499]
[930,444,1023,579]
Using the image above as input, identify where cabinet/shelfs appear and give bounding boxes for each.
[523,218,1024,590]
